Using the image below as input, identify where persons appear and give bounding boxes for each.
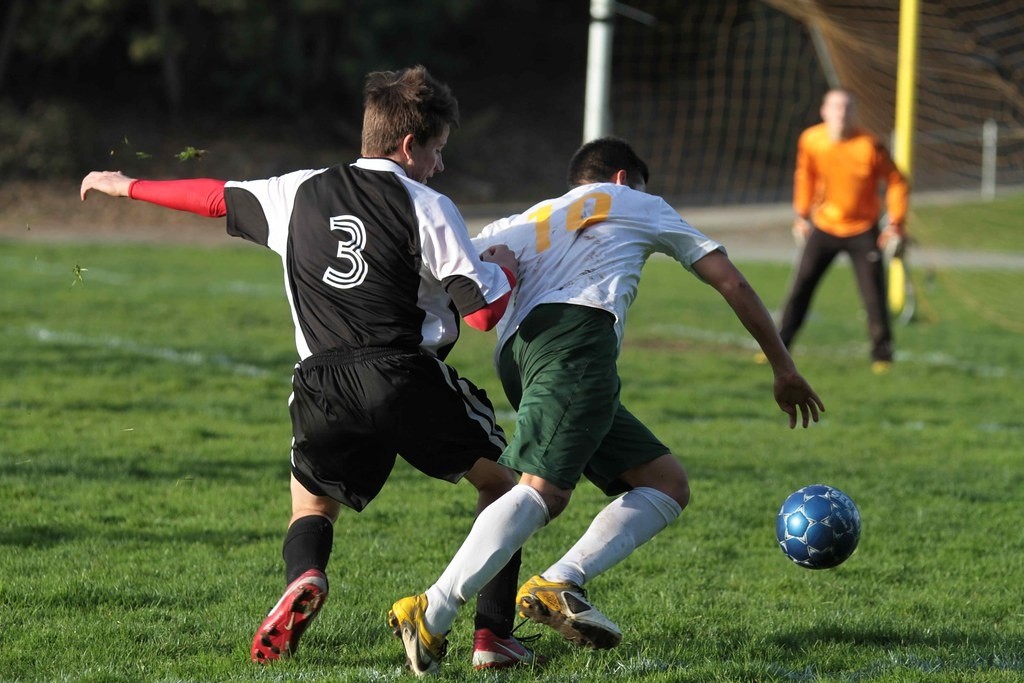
[79,66,552,671]
[753,88,912,373]
[388,136,826,678]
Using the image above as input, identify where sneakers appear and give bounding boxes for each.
[251,569,328,665]
[388,594,452,680]
[517,574,622,650]
[473,617,550,671]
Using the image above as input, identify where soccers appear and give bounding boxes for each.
[776,484,862,570]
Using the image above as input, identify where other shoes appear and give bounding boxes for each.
[873,361,891,374]
[753,353,768,366]
